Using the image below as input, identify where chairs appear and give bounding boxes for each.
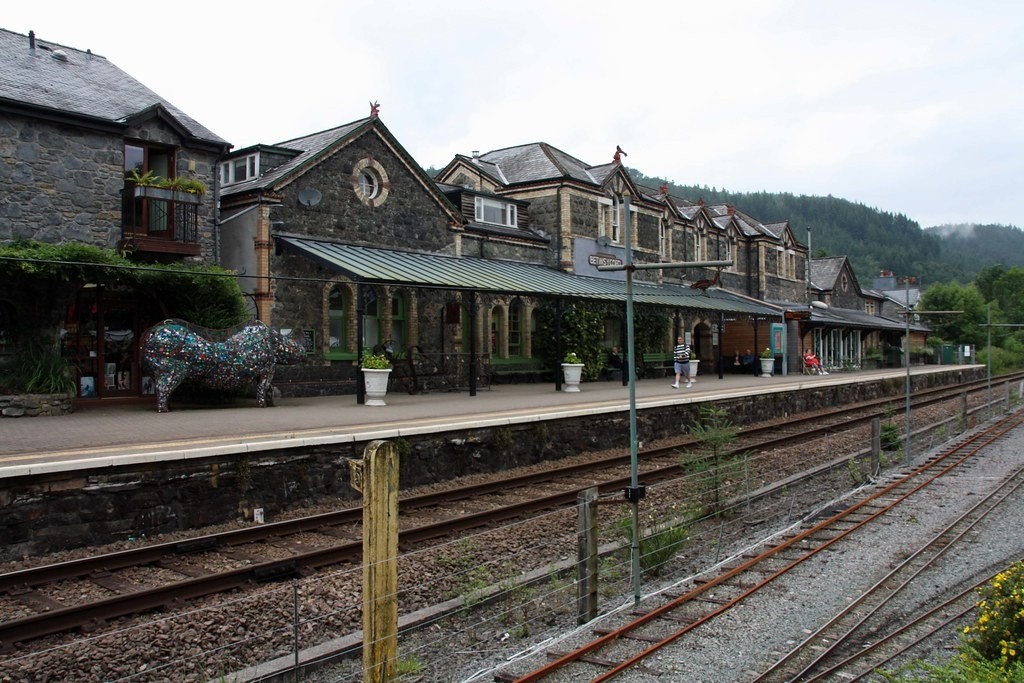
[802,357,818,376]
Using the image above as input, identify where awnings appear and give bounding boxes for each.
[270,231,602,298]
[551,269,786,320]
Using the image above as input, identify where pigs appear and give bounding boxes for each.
[138,319,307,413]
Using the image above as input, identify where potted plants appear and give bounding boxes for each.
[684,352,701,383]
[124,167,205,205]
[561,353,585,392]
[863,345,932,369]
[361,354,392,406]
[759,348,775,377]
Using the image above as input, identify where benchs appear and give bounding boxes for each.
[643,352,677,379]
[490,358,552,384]
[598,353,641,381]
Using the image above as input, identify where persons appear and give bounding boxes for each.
[804,348,830,375]
[78,362,155,399]
[608,346,625,370]
[671,336,693,389]
[728,348,761,376]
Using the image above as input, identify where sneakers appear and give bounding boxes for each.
[671,382,679,389]
[684,381,692,388]
